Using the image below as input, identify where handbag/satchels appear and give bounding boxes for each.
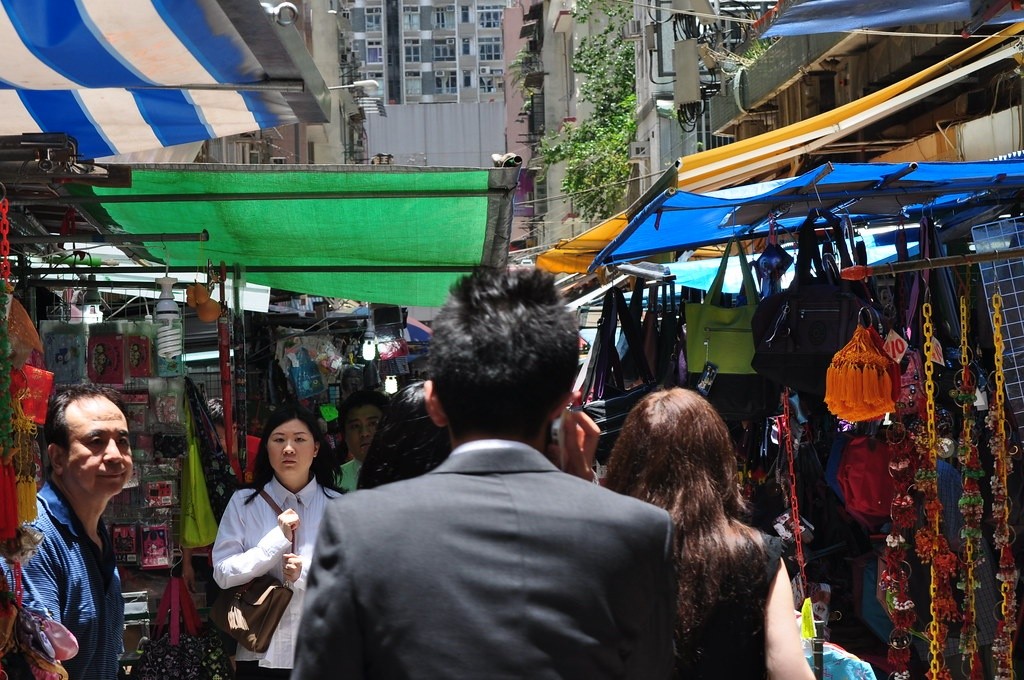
[168,375,242,549]
[206,573,294,653]
[576,206,1006,646]
[128,576,236,680]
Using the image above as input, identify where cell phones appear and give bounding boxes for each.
[553,402,570,475]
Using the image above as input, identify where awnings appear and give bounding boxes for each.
[0,0,330,164]
[758,2,1023,39]
[538,19,1024,296]
[53,154,521,307]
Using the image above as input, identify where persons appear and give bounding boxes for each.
[357,380,451,490]
[1,380,133,679]
[212,403,342,680]
[326,390,388,492]
[291,264,673,679]
[605,387,815,679]
